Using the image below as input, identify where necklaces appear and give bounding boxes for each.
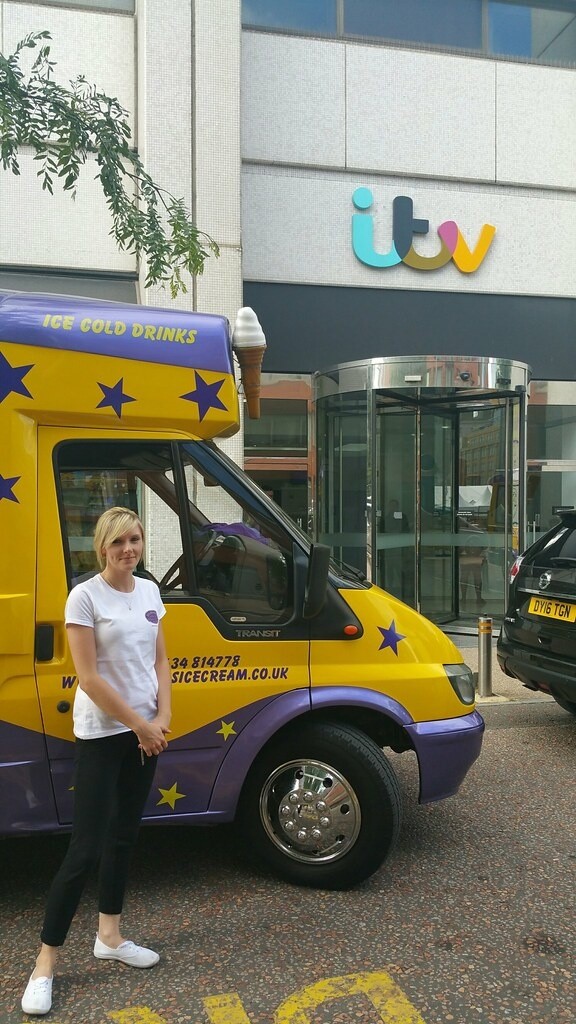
[105,575,134,611]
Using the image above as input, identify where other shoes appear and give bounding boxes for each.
[460,598,467,604]
[476,599,487,605]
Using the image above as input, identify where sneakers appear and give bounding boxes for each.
[20,966,53,1015]
[93,931,160,968]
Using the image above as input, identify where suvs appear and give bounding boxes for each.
[495,504,576,714]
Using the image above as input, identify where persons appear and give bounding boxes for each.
[386,499,409,534]
[22,507,172,1014]
[459,513,488,604]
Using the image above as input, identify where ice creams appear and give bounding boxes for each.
[232,306,266,420]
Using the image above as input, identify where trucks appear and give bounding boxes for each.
[1,286,487,891]
